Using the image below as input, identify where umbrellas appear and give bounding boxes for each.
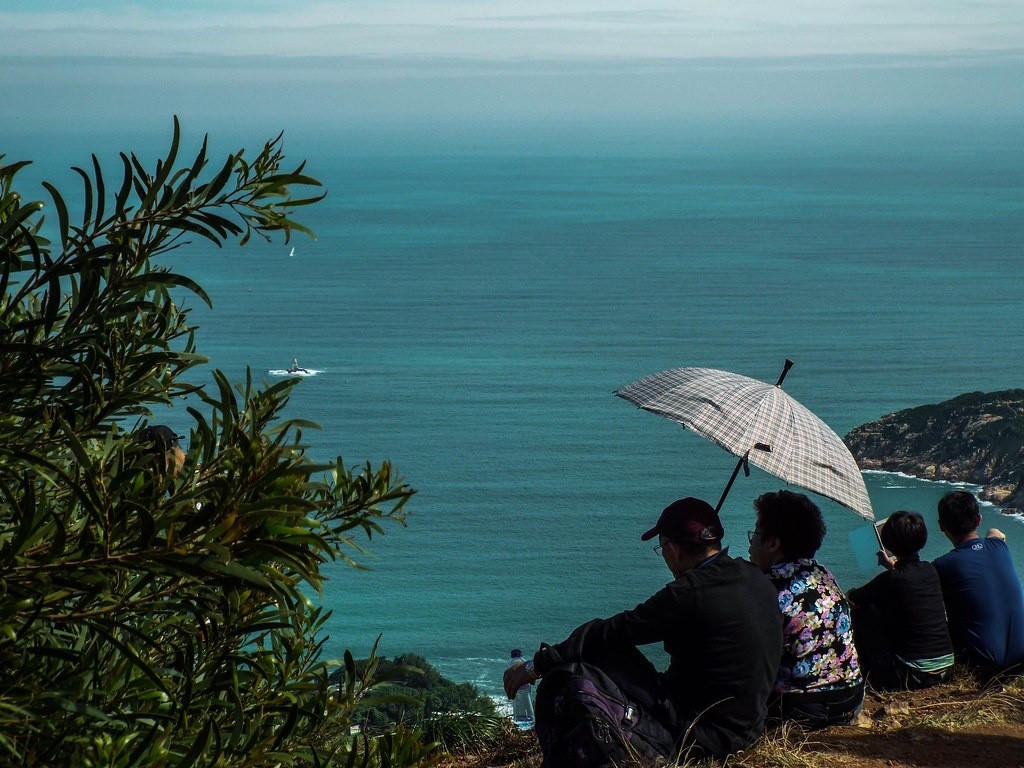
[613,356,877,523]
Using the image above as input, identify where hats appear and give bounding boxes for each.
[641,497,725,545]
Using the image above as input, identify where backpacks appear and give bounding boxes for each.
[534,642,680,768]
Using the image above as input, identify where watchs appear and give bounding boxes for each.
[521,661,537,679]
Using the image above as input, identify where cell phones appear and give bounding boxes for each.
[873,517,898,562]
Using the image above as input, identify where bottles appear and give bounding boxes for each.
[508,649,535,730]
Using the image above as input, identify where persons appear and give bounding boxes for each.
[502,498,784,757]
[847,491,1024,689]
[748,491,864,724]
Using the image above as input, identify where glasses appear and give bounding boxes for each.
[653,539,672,556]
[748,530,762,541]
